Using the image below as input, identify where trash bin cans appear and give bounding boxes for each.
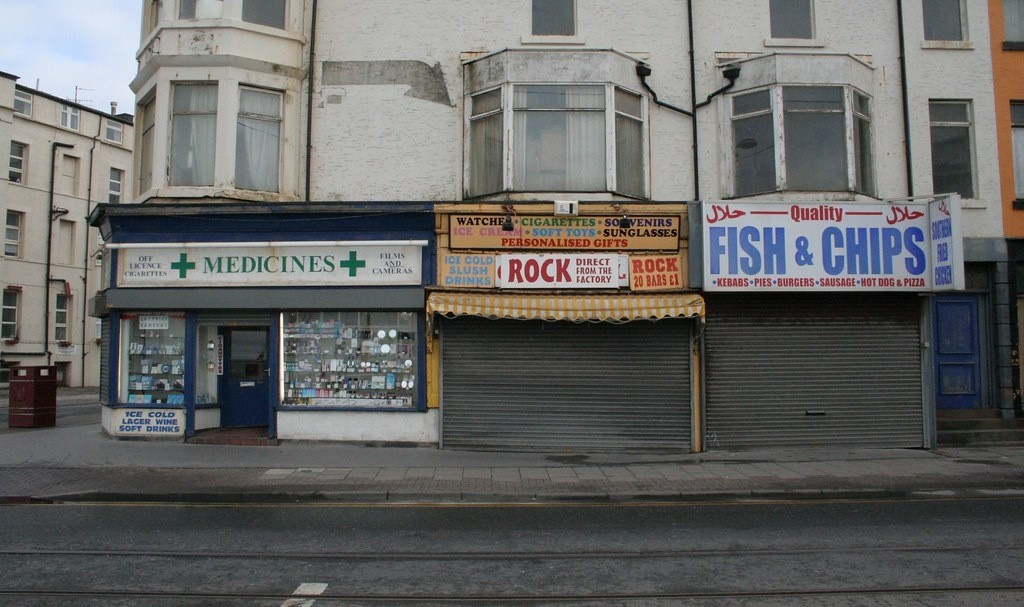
[8,365,58,428]
